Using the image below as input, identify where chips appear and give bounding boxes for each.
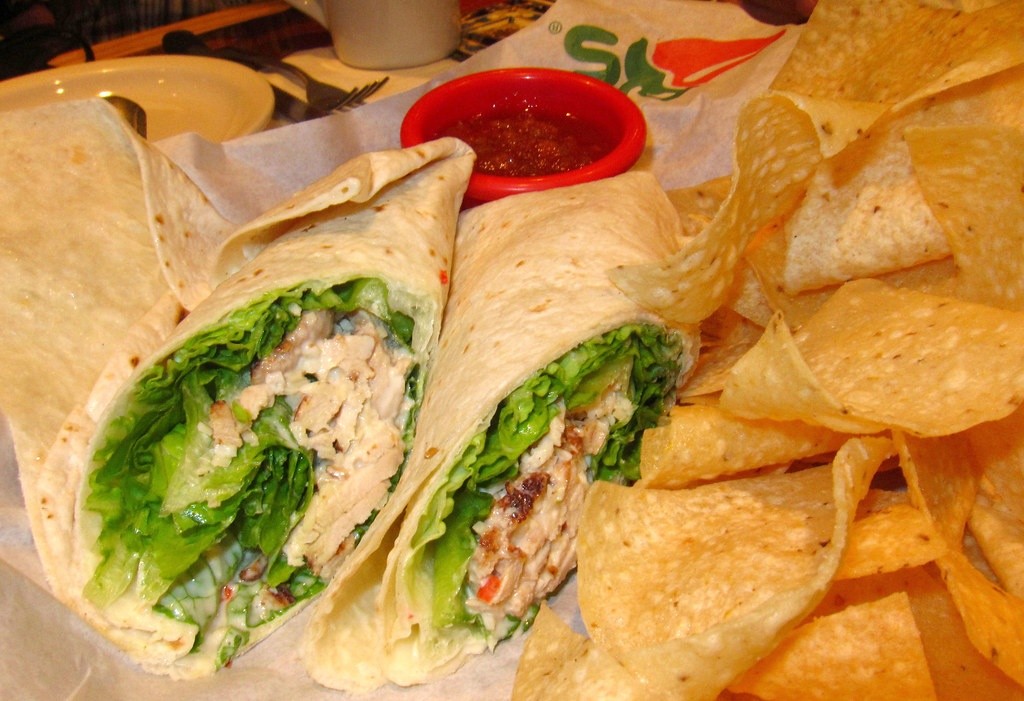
[510,0,1024,701]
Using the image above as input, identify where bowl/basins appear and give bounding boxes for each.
[399,67,648,205]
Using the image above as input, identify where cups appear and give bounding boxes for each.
[290,0,463,71]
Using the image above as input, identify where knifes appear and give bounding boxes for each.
[164,30,333,125]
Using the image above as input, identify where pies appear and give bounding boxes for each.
[1,95,698,690]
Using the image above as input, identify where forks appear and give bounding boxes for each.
[215,41,389,113]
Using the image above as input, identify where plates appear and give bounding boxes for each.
[0,52,277,174]
